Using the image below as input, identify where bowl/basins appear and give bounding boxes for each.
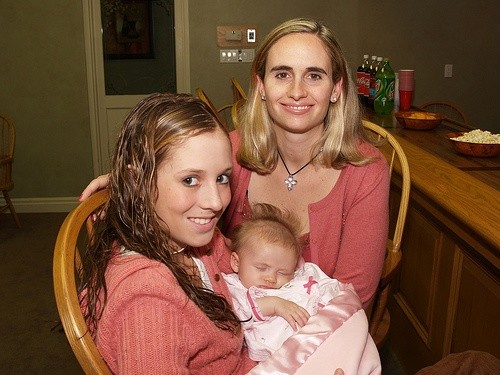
[446,131,500,157]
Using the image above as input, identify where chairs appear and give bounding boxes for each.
[420,99,469,125]
[361,120,410,350]
[0,112,22,231]
[196,78,248,133]
[53,188,110,375]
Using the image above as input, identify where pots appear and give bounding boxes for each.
[393,111,448,130]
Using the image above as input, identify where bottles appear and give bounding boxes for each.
[369,57,384,111]
[374,57,396,115]
[357,54,371,106]
[363,55,378,108]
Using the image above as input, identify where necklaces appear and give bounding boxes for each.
[277,147,322,191]
[170,245,188,254]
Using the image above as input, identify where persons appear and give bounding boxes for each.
[79,17,390,310]
[220,203,349,361]
[81,92,500,375]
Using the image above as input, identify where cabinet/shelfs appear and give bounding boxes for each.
[357,105,500,375]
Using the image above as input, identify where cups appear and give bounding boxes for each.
[395,69,415,110]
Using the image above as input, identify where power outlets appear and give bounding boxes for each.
[444,63,453,77]
